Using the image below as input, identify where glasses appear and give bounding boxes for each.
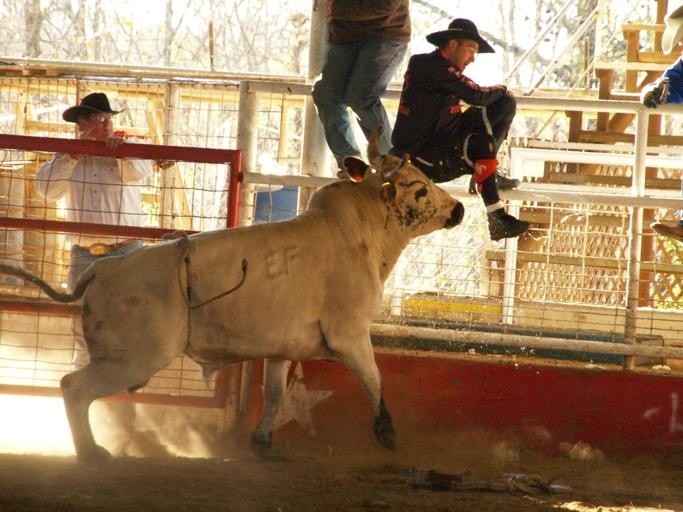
[91,114,114,122]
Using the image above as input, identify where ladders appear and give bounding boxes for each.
[500,0,641,140]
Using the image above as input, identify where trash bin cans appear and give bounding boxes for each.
[253,184,296,223]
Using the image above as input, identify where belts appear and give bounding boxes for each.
[79,238,134,256]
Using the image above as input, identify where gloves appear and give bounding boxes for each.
[642,87,662,108]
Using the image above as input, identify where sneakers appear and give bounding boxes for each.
[488,213,529,240]
[468,171,521,195]
[650,222,682,242]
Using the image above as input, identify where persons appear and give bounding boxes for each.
[32,90,150,386]
[389,16,530,244]
[308,0,411,180]
[639,5,682,240]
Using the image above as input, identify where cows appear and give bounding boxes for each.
[0,122,465,469]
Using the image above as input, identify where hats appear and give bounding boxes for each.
[661,6,683,55]
[426,18,496,54]
[62,92,126,122]
